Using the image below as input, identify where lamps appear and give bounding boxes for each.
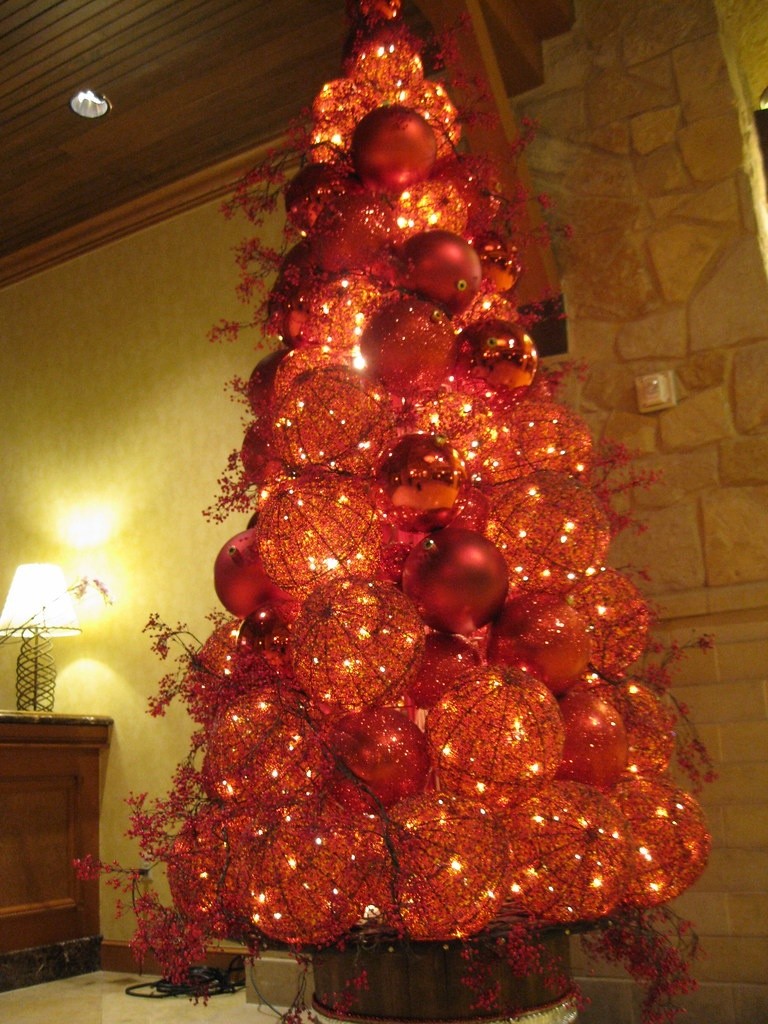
[0,562,84,713]
[69,93,112,120]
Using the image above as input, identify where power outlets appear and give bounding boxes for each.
[139,868,152,880]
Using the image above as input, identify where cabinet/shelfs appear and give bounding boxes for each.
[0,713,113,993]
